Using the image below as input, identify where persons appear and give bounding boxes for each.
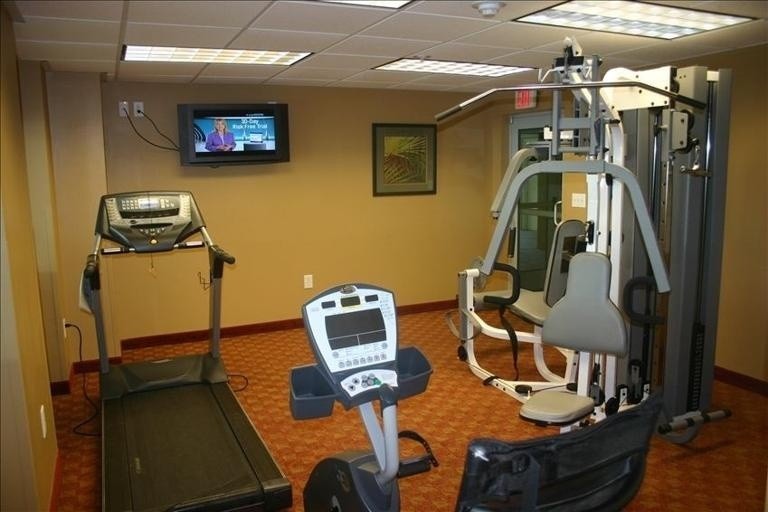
[205,119,236,152]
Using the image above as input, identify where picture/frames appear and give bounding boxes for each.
[372,123,437,196]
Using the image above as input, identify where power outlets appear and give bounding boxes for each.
[61,316,68,339]
[134,100,145,120]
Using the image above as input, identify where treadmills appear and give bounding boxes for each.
[78,190,293,511]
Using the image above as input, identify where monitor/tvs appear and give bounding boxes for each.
[178,102,290,165]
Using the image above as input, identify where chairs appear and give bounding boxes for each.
[518,252,631,429]
[506,220,585,326]
